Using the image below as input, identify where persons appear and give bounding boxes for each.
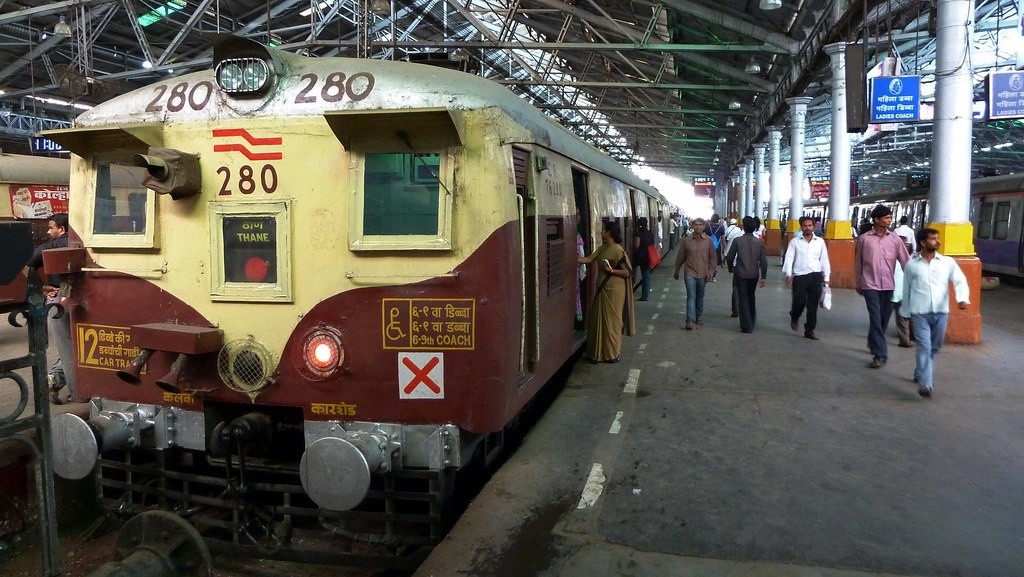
[856,205,972,397]
[658,212,822,282]
[577,221,634,364]
[13,185,34,218]
[574,207,587,330]
[728,218,767,334]
[782,216,832,340]
[674,218,717,330]
[21,213,88,405]
[635,217,654,301]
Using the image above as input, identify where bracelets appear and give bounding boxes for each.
[611,269,613,273]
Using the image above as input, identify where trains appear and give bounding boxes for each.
[762,172,1024,287]
[0,148,150,313]
[45,33,687,549]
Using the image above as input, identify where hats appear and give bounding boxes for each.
[730,218,737,224]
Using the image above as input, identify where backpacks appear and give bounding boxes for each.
[708,224,722,249]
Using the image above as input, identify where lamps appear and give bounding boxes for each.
[710,0,782,174]
[448,38,470,62]
[54,14,72,37]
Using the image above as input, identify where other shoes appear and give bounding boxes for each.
[685,314,704,330]
[913,368,934,398]
[731,312,738,318]
[739,328,755,334]
[899,340,914,347]
[868,341,887,368]
[804,330,820,340]
[791,315,801,331]
[48,374,63,405]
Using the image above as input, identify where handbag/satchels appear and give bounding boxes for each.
[648,244,662,268]
[819,284,833,310]
